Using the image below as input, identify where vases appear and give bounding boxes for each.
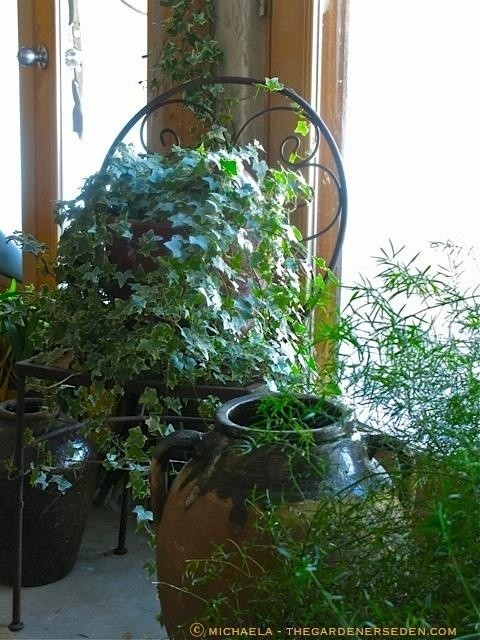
[0,389,105,590]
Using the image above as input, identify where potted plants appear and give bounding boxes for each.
[0,141,339,562]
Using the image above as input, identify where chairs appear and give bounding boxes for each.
[0,72,350,631]
